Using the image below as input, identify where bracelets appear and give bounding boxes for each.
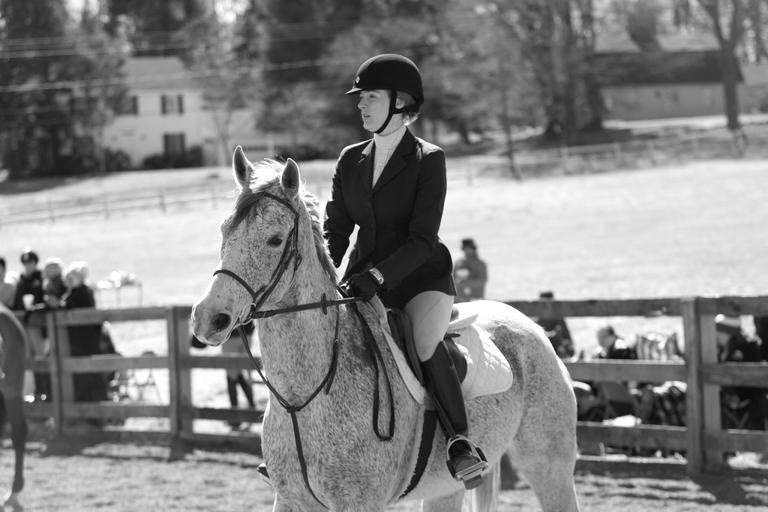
[368,267,384,286]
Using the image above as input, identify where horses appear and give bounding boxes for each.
[188,143,582,512]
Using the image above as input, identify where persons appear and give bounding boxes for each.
[454,238,487,297]
[27,262,67,308]
[530,285,767,457]
[321,52,489,479]
[14,250,48,402]
[222,320,256,429]
[0,258,15,310]
[61,267,109,425]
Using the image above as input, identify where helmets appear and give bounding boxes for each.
[344,53,424,105]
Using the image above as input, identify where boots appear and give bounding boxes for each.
[421,339,484,490]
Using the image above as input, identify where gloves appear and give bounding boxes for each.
[346,270,381,303]
